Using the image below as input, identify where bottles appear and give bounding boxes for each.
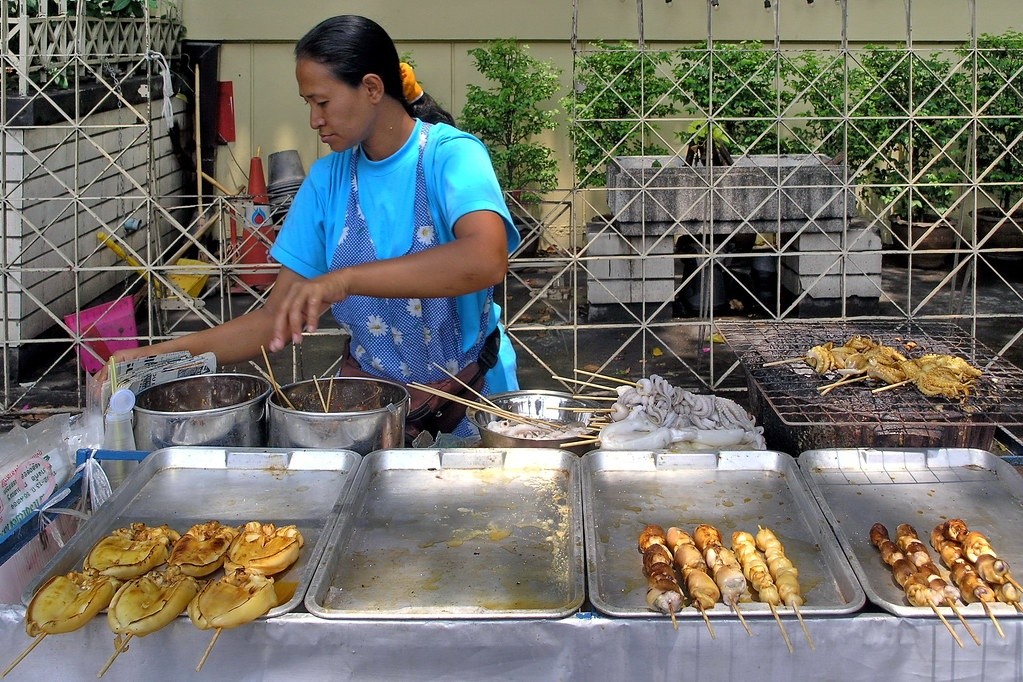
[100,390,138,492]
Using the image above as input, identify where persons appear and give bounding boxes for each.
[91,13,523,441]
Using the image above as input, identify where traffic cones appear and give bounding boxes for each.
[224,157,281,295]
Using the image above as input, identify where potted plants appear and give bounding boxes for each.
[862,23,1022,267]
[458,38,559,257]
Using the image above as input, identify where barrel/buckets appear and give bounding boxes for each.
[134,373,271,456]
[269,377,412,454]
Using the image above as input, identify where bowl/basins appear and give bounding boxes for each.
[467,390,605,455]
